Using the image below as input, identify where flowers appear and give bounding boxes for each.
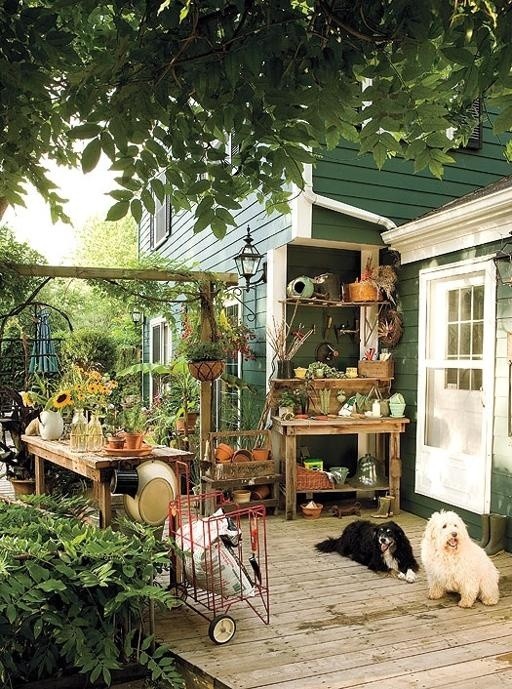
[19,356,120,413]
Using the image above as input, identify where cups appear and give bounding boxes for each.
[292,368,306,377]
[345,367,357,378]
[108,462,138,500]
[330,467,349,486]
[389,393,406,418]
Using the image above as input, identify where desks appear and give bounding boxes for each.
[22,430,194,583]
[271,414,412,520]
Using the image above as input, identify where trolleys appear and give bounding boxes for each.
[163,461,271,644]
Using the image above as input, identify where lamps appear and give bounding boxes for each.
[130,305,145,328]
[234,223,267,294]
[493,231,512,287]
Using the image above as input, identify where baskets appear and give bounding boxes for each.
[295,465,337,491]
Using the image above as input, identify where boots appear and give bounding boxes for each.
[369,496,396,518]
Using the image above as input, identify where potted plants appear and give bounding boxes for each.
[276,390,295,418]
[103,402,149,449]
[179,338,231,382]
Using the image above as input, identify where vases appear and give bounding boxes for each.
[389,403,406,416]
[299,501,323,520]
[276,360,295,378]
[175,406,200,432]
[37,409,63,440]
[85,415,102,453]
[390,393,405,404]
[212,442,270,503]
[69,409,88,452]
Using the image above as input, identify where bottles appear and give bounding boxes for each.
[252,487,270,499]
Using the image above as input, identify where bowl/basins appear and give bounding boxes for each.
[232,489,251,503]
[342,284,377,301]
[124,461,178,525]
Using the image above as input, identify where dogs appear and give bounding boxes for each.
[314,519,420,583]
[420,508,501,608]
